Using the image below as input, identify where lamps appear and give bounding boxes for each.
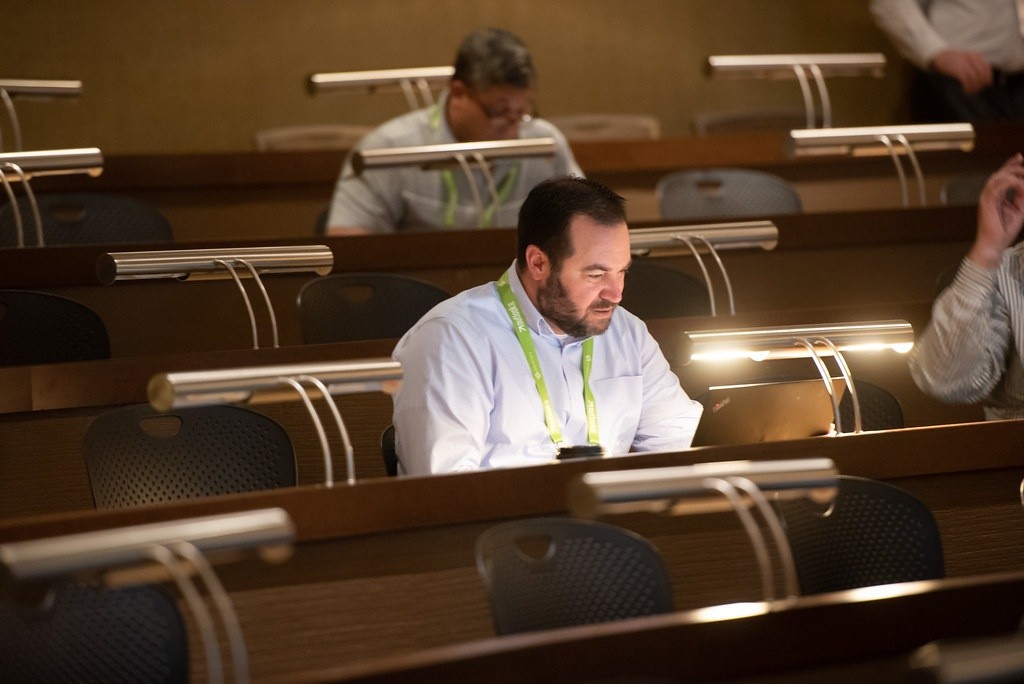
[701,51,888,129]
[782,124,975,208]
[580,457,839,603]
[0,79,85,151]
[150,356,405,486]
[97,245,334,351]
[668,311,915,435]
[0,506,295,684]
[630,220,778,317]
[304,66,457,113]
[0,147,105,247]
[353,136,556,228]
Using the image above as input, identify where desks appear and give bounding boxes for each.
[0,418,1024,684]
[0,118,1024,206]
[0,302,981,515]
[315,572,1024,684]
[0,208,981,357]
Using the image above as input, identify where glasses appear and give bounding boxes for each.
[466,88,541,130]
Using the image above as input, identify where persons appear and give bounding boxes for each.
[392,176,703,481]
[325,27,588,236]
[906,152,1024,421]
[866,1,1024,124]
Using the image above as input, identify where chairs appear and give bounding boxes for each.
[253,124,370,151]
[652,169,804,220]
[0,190,174,249]
[620,262,712,319]
[0,577,191,684]
[0,289,113,367]
[80,404,297,509]
[474,518,672,636]
[555,115,662,142]
[296,272,452,345]
[782,474,946,597]
[692,376,904,433]
